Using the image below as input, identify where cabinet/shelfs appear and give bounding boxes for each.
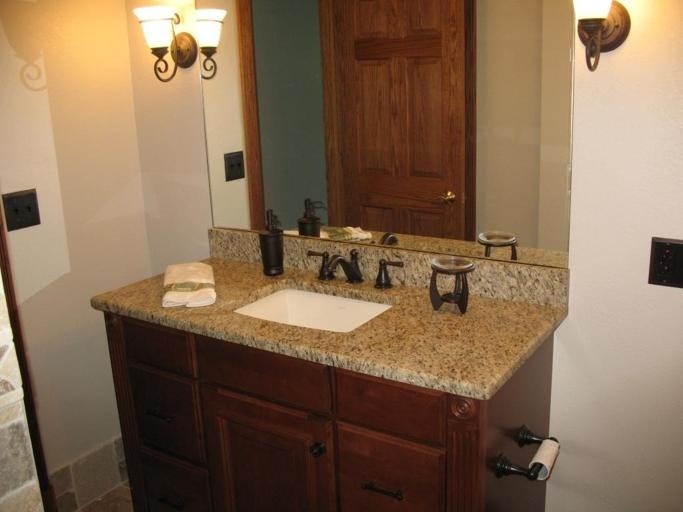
[331,326,553,512]
[194,337,338,512]
[113,313,211,512]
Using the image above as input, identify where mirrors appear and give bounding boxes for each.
[195,1,573,270]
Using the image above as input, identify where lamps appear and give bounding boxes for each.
[129,4,197,84]
[571,0,631,74]
[195,8,229,82]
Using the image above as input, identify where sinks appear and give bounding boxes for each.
[231,287,392,333]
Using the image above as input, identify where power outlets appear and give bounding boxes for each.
[646,237,683,290]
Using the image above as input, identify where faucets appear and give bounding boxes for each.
[326,247,363,285]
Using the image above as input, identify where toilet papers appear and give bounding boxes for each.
[528,438,560,481]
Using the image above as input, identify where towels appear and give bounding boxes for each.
[320,227,372,243]
[161,263,216,308]
